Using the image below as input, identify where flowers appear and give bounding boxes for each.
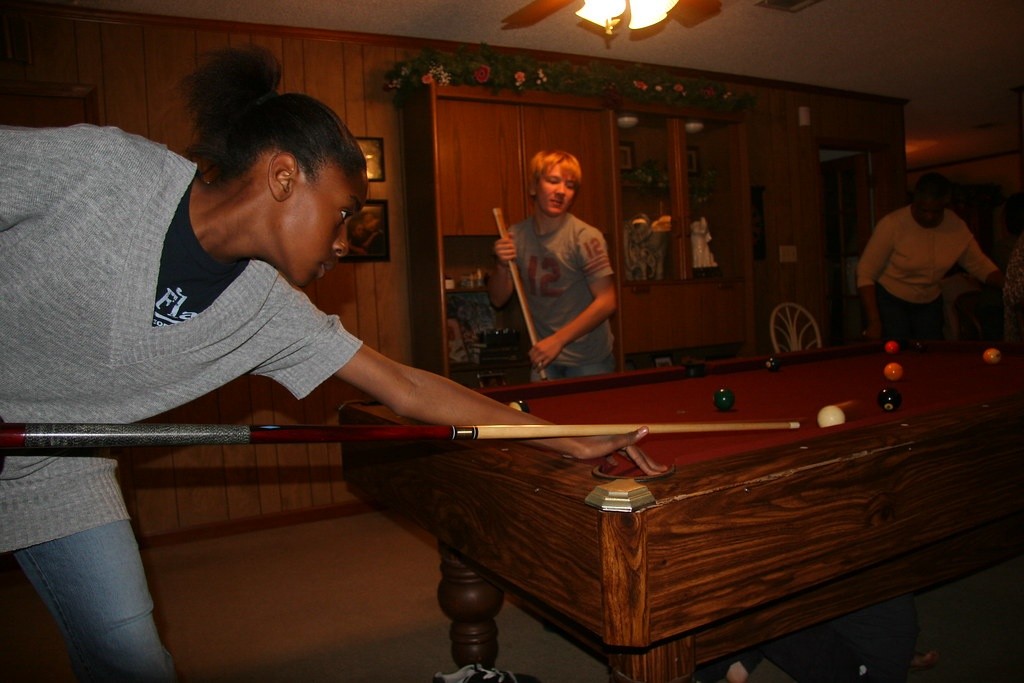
[381,34,740,118]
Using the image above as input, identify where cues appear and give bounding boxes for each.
[1,417,803,453]
[492,206,546,380]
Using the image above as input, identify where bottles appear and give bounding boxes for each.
[444,274,455,289]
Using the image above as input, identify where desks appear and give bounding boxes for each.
[335,340,1024,683]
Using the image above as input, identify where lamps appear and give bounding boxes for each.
[684,119,705,135]
[617,112,640,130]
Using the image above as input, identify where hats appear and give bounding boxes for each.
[915,173,953,212]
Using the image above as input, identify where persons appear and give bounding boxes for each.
[853,174,1007,341]
[487,148,618,382]
[0,43,669,683]
[1001,192,1024,343]
[695,590,940,683]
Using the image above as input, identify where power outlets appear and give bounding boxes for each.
[780,245,798,263]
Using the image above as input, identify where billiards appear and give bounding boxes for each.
[884,340,900,354]
[713,386,735,411]
[982,347,1001,364]
[883,362,904,381]
[876,387,902,412]
[509,399,530,414]
[766,356,780,371]
[817,404,846,429]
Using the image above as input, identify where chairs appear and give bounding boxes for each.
[769,301,822,354]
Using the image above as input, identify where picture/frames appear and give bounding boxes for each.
[0,77,102,127]
[354,137,385,182]
[687,145,703,178]
[338,199,391,263]
[619,141,636,175]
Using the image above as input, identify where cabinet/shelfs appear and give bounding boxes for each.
[402,76,758,378]
